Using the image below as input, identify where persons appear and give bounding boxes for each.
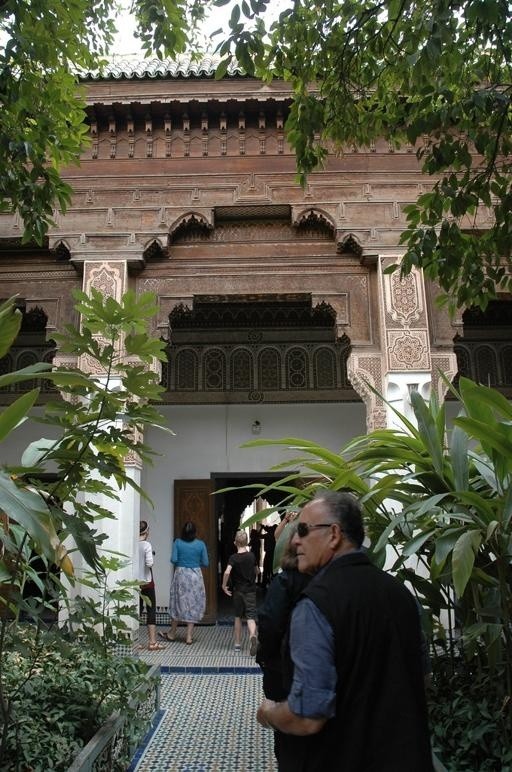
[248,524,280,588]
[270,501,301,578]
[255,489,437,771]
[221,530,261,652]
[253,517,316,764]
[139,519,167,651]
[159,519,210,645]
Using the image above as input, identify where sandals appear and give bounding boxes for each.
[148,629,193,650]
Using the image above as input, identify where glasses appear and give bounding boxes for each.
[297,521,331,537]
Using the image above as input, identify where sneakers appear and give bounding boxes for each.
[234,632,258,657]
